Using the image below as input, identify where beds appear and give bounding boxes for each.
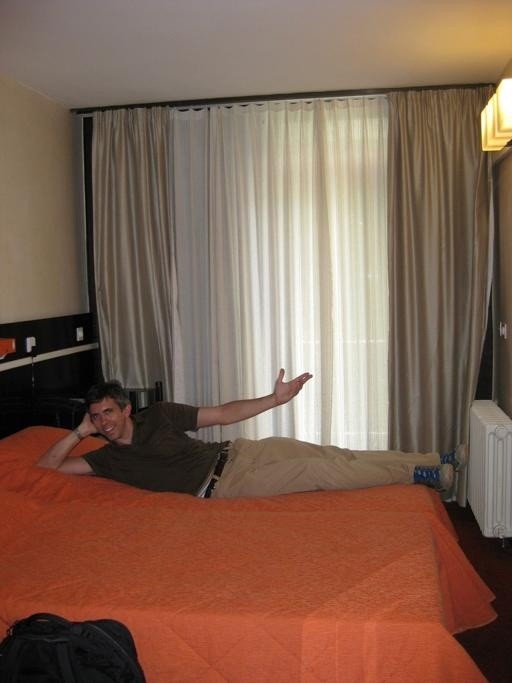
[1,426,498,683]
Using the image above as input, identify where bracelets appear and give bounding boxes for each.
[73,429,83,441]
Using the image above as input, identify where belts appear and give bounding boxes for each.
[203,441,230,498]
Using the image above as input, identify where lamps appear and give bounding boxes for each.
[478,78,512,152]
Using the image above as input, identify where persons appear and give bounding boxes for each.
[35,368,470,500]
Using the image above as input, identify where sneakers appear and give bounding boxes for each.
[439,444,467,472]
[413,463,454,492]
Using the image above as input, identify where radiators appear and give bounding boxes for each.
[466,399,512,540]
